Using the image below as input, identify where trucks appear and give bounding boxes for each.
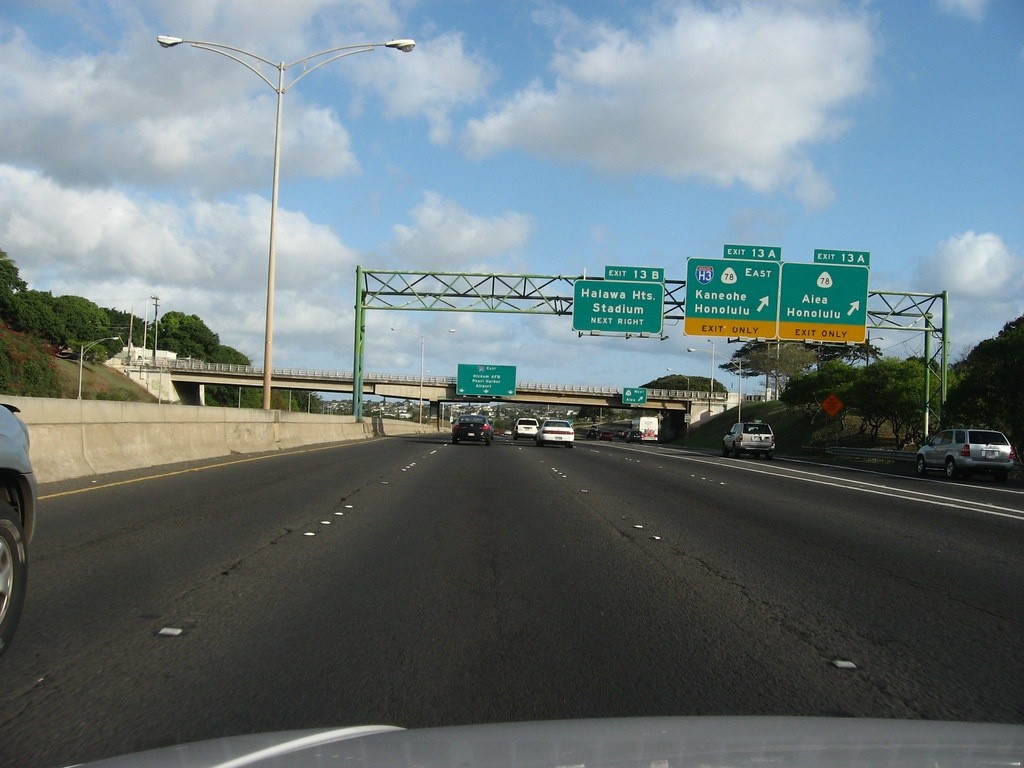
[626,417,659,441]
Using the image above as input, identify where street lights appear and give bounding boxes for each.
[156,35,416,408]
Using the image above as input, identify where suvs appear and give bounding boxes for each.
[512,418,540,441]
[916,427,1016,481]
[722,422,777,459]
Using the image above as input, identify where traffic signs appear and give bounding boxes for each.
[684,255,781,340]
[779,261,870,343]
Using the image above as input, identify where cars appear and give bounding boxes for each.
[536,420,574,449]
[587,425,599,439]
[627,431,645,444]
[451,415,492,447]
[598,430,613,441]
